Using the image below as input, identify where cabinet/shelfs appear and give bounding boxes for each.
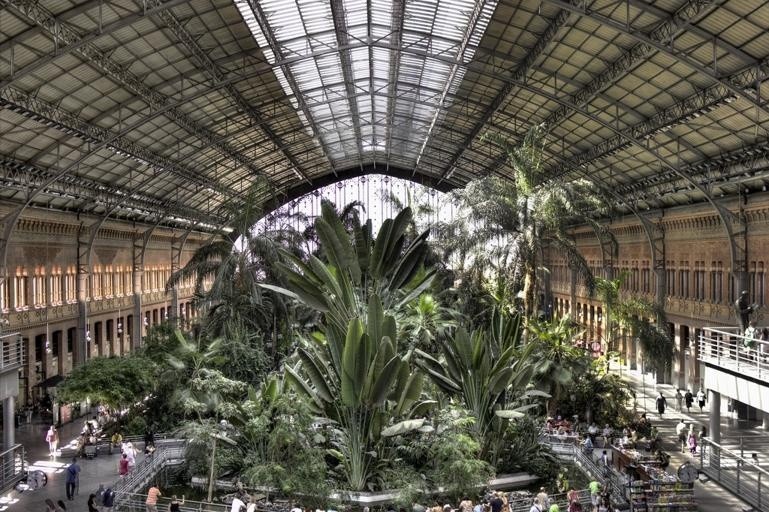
[630,483,694,512]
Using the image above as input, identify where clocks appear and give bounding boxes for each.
[678,462,697,483]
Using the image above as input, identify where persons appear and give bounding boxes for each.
[700,426,707,459]
[677,419,687,453]
[528,415,663,512]
[42,387,186,512]
[733,290,769,363]
[231,494,258,512]
[426,488,512,512]
[655,393,668,419]
[289,502,370,512]
[689,424,698,454]
[675,388,706,414]
